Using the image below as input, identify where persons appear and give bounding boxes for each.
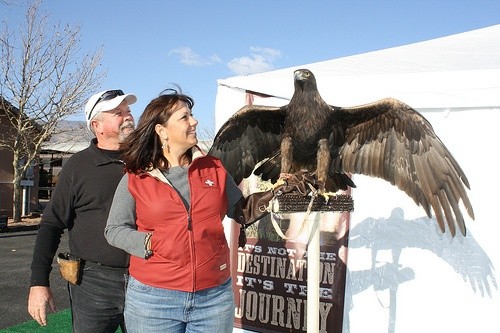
[104,79,316,332]
[26,88,138,332]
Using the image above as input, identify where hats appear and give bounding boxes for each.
[85,90,137,132]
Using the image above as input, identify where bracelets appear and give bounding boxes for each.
[143,232,155,258]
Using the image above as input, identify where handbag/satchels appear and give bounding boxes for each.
[57,252,81,285]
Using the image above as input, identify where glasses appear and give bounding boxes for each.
[88,89,124,120]
